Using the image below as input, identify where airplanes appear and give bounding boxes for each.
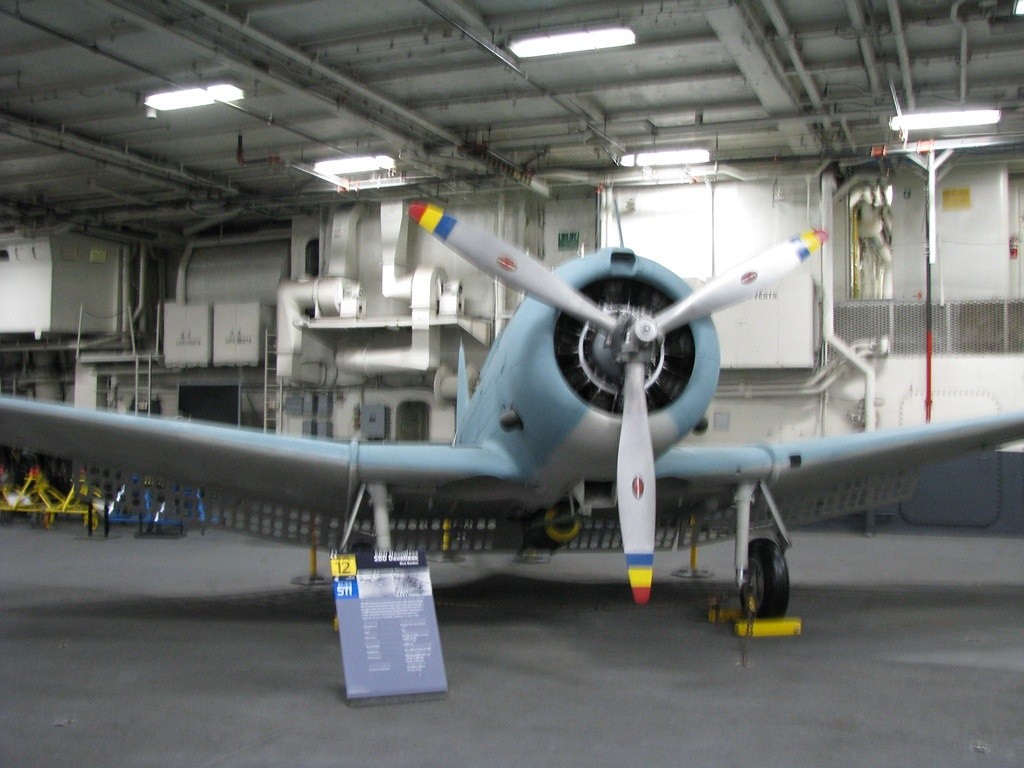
[1,199,1024,624]
